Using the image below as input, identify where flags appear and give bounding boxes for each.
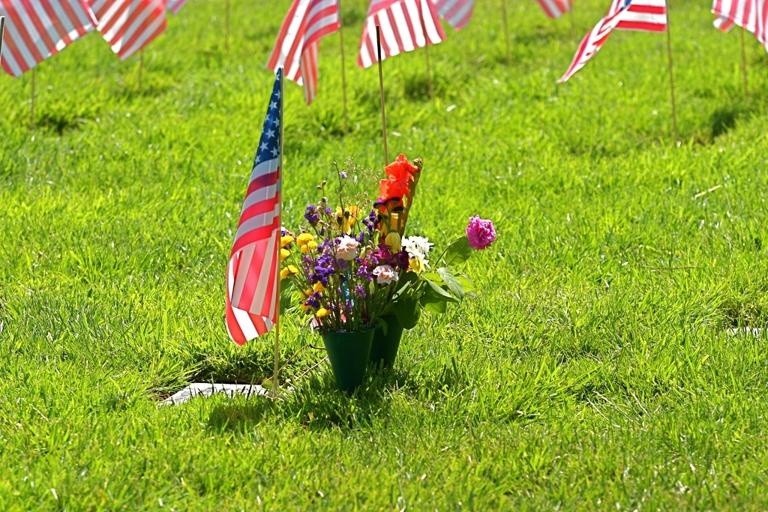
[0,0,90,81]
[265,0,343,110]
[707,2,767,65]
[432,1,482,33]
[356,0,446,76]
[76,0,186,63]
[221,61,287,345]
[554,0,666,85]
[535,0,578,20]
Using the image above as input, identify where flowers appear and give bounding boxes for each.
[357,151,502,334]
[271,155,458,338]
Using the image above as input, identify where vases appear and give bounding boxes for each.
[359,311,409,383]
[314,319,378,399]
